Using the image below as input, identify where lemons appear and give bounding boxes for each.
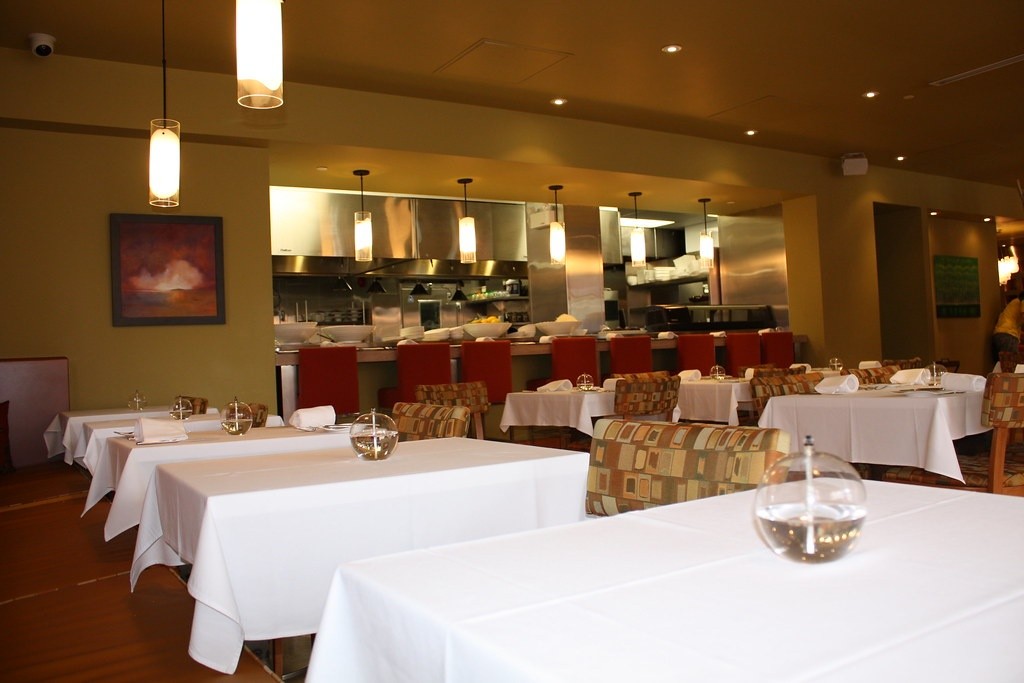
[471,315,502,324]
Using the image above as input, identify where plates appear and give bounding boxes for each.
[400,323,462,341]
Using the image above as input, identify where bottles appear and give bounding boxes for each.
[710,365,725,381]
[751,435,868,566]
[576,373,593,391]
[828,357,843,371]
[220,396,253,436]
[128,390,147,410]
[169,395,192,421]
[349,408,399,461]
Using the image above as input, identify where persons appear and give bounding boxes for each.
[992,292,1024,358]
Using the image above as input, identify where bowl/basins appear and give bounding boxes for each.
[274,321,317,345]
[323,325,375,343]
[463,323,512,340]
[535,321,585,336]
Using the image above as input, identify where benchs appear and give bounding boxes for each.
[841,365,901,385]
[392,402,470,442]
[614,376,681,422]
[749,372,825,416]
[415,381,489,441]
[879,357,921,370]
[885,374,1024,497]
[586,420,791,516]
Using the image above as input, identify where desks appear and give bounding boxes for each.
[758,388,993,484]
[74,416,283,460]
[501,389,680,435]
[82,425,359,543]
[304,480,1024,683]
[43,409,219,465]
[680,379,751,426]
[129,439,589,675]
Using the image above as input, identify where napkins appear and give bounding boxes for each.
[814,376,858,394]
[790,364,812,373]
[745,368,754,378]
[890,369,929,385]
[859,361,882,368]
[290,405,336,427]
[603,379,624,391]
[943,373,987,393]
[679,369,701,380]
[131,417,185,444]
[537,380,572,391]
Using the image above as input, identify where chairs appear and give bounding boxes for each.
[298,347,359,415]
[609,336,652,373]
[378,343,452,409]
[461,340,512,404]
[527,337,601,389]
[762,332,794,368]
[676,335,716,377]
[726,333,761,375]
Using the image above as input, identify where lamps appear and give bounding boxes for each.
[367,278,387,294]
[150,0,181,207]
[549,185,566,265]
[409,281,429,296]
[700,199,714,269]
[451,281,469,302]
[236,0,285,111]
[458,178,477,264]
[628,192,646,268]
[352,169,373,262]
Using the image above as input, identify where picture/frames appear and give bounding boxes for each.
[110,213,226,328]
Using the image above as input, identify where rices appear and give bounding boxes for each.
[554,314,577,322]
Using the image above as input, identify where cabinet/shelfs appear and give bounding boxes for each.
[473,295,532,328]
